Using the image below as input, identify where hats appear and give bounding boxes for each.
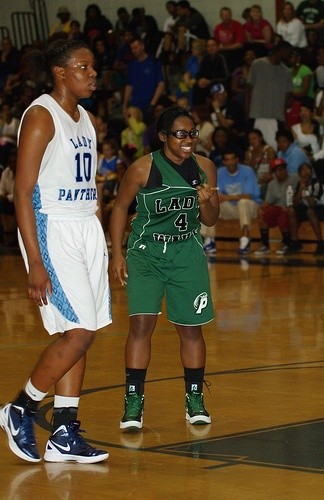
[269,159,288,174]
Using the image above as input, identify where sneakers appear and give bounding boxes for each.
[0,401,42,462]
[204,237,216,253]
[239,236,250,255]
[44,420,110,463]
[120,395,145,431]
[185,391,210,425]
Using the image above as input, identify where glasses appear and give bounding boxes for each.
[164,130,199,139]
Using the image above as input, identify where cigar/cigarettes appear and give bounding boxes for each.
[209,187,220,191]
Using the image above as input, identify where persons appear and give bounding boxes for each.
[0,0,81,249]
[283,163,324,256]
[203,149,263,256]
[253,158,298,256]
[0,32,112,464]
[110,105,220,431]
[161,0,324,199]
[78,0,174,247]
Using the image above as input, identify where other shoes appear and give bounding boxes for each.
[254,245,270,254]
[315,242,324,256]
[275,246,287,254]
[284,242,303,255]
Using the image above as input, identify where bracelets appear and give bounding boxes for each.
[215,107,220,114]
